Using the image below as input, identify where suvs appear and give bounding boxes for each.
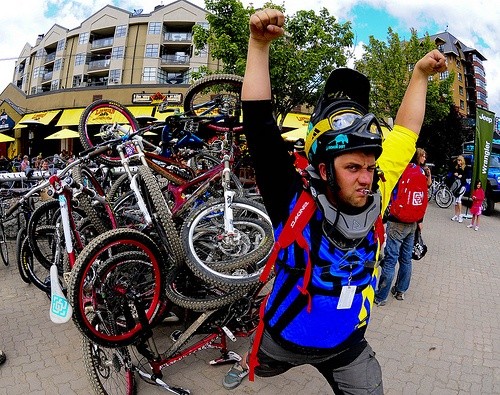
[449,153,500,215]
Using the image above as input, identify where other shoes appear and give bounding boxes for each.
[391,286,404,300]
[459,218,463,222]
[374,299,385,306]
[451,215,458,221]
[474,226,479,231]
[466,224,473,228]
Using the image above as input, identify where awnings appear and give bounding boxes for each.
[54,106,155,127]
[276,113,311,128]
[13,109,62,129]
[154,105,243,123]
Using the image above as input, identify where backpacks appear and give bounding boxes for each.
[450,179,466,198]
[390,164,428,223]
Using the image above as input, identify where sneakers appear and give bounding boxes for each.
[223,361,249,390]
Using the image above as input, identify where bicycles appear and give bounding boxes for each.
[428,177,454,208]
[0,74,276,395]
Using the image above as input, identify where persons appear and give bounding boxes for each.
[450,155,471,223]
[221,8,448,395]
[373,151,424,307]
[0,150,76,171]
[467,180,485,231]
[416,146,432,229]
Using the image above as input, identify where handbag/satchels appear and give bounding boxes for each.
[412,228,427,261]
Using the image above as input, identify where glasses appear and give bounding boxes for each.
[327,106,365,130]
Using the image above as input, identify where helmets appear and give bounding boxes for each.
[305,100,384,168]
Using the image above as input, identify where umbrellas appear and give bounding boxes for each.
[0,133,15,143]
[44,129,81,151]
[281,126,309,143]
